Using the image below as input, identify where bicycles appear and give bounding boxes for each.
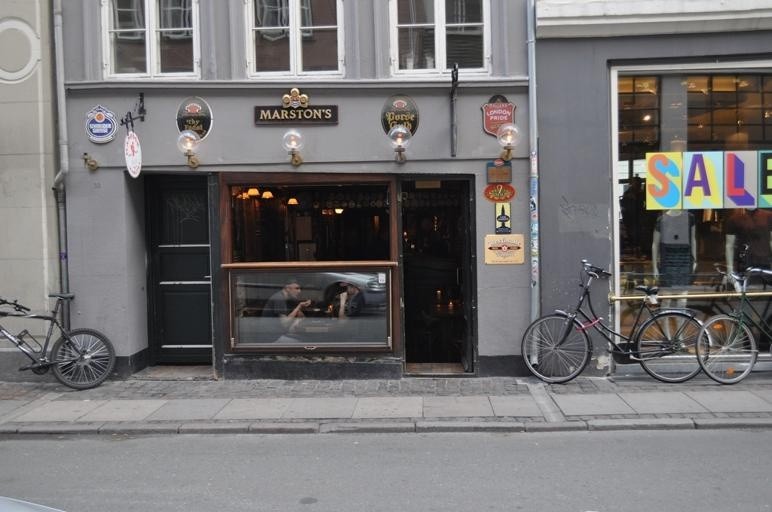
[697,258,771,388]
[516,259,713,387]
[0,292,118,393]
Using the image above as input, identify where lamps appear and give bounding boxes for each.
[386,125,412,164]
[282,131,305,167]
[176,129,202,168]
[496,123,522,161]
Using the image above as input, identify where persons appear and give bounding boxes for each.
[650,206,697,351]
[621,176,646,257]
[723,208,772,354]
[255,275,312,343]
[332,278,364,341]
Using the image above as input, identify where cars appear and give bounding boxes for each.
[233,268,387,319]
